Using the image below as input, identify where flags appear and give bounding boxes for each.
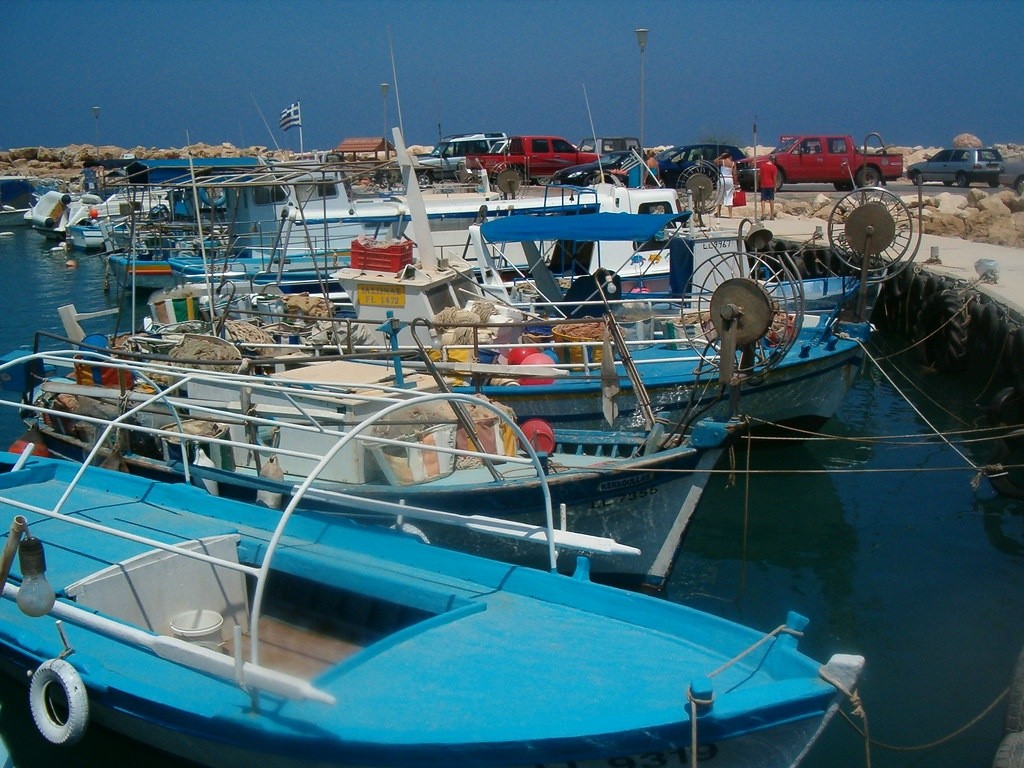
[280,102,301,132]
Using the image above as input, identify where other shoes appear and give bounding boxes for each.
[761,215,767,221]
[770,217,774,221]
[714,213,718,218]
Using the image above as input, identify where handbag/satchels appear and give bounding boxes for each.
[733,191,746,207]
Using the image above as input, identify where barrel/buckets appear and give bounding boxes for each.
[79,334,110,361]
[520,418,554,456]
[169,609,226,654]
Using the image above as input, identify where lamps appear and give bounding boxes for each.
[738,218,774,277]
[437,259,449,272]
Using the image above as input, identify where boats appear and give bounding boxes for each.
[0,349,867,768]
[0,29,893,597]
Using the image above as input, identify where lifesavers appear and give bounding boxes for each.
[910,288,968,372]
[28,657,90,748]
[774,240,830,276]
[894,262,1024,395]
[199,188,227,206]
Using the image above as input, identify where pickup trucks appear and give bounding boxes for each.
[735,133,904,192]
[466,135,603,186]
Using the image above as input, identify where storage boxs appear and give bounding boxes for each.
[515,291,540,302]
[660,321,722,350]
[755,314,795,348]
[615,317,655,351]
[351,239,413,273]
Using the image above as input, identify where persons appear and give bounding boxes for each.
[644,153,659,189]
[758,155,778,221]
[713,149,738,219]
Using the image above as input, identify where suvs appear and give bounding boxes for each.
[409,131,510,188]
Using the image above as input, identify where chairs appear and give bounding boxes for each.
[813,145,820,153]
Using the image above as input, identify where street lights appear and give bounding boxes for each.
[379,82,392,192]
[92,106,101,191]
[634,28,650,190]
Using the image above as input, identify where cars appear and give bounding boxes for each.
[551,150,651,188]
[640,142,749,189]
[906,147,1005,188]
[997,157,1024,196]
